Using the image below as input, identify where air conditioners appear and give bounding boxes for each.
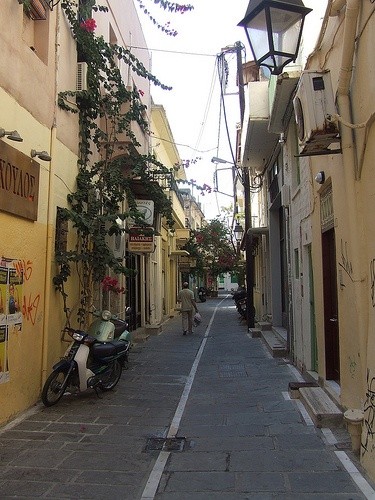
[292,71,340,154]
[100,221,125,258]
[77,62,88,90]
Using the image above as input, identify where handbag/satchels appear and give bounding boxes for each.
[193,312,203,328]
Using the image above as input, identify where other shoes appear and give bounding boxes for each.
[183,330,187,335]
[188,331,195,335]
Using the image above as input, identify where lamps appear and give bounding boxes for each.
[31,150,52,161]
[234,222,244,244]
[237,0,313,75]
[211,157,235,165]
[0,128,24,142]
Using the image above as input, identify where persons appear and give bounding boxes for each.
[177,282,199,335]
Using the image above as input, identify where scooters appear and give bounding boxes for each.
[231,283,256,323]
[195,284,208,303]
[41,327,131,408]
[79,307,133,357]
